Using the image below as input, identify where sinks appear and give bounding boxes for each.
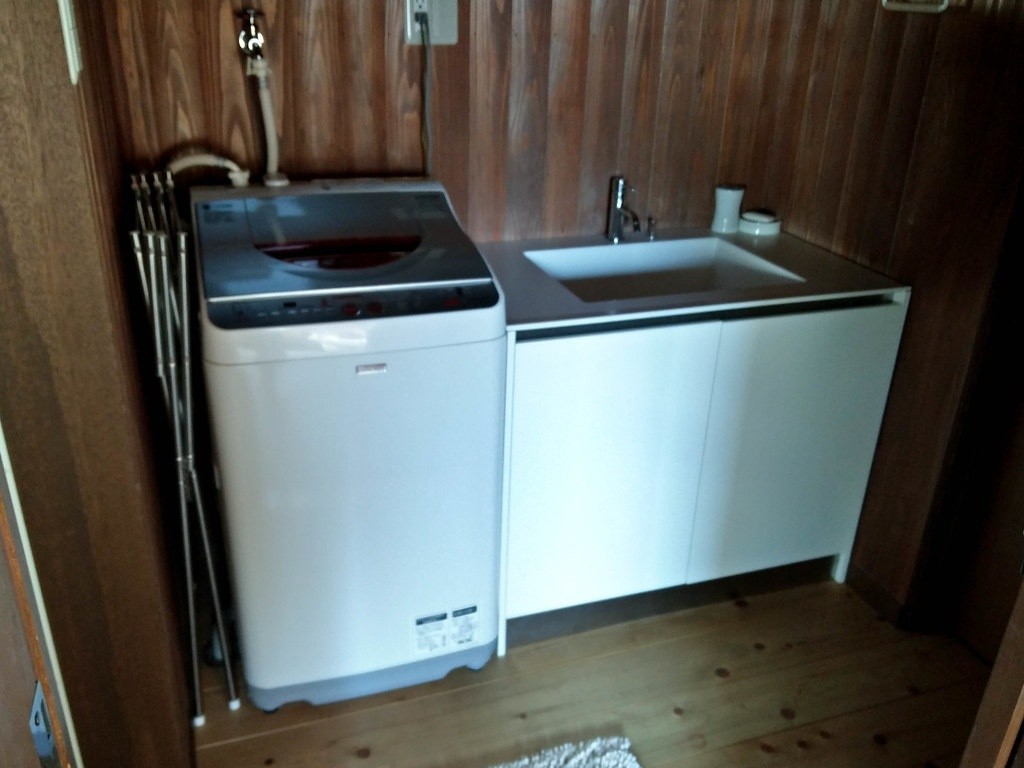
[523,228,810,310]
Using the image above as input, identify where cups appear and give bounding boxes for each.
[710,185,744,234]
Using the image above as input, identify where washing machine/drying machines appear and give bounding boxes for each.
[188,171,513,722]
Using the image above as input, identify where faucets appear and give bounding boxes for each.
[606,177,644,242]
[237,7,268,67]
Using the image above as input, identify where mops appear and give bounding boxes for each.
[480,735,644,767]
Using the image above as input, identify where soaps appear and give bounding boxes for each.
[742,211,776,222]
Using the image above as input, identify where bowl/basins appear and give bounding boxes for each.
[739,211,782,235]
